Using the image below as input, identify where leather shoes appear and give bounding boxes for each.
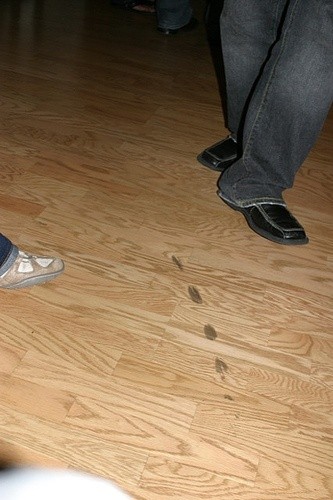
[196,138,244,172]
[221,199,309,246]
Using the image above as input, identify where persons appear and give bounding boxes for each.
[122,0,333,245]
[0,233,65,289]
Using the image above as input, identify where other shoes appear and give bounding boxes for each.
[159,18,198,37]
[0,251,65,289]
